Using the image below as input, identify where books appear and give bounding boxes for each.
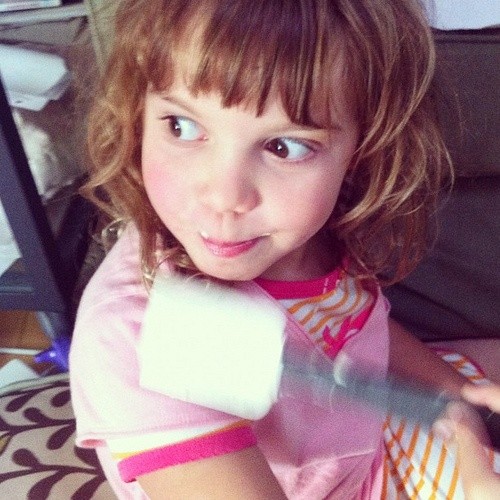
[0,0,90,46]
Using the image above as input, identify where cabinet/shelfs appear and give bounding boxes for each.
[0,0,119,371]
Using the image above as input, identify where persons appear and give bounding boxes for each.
[68,0,500,500]
[431,382,500,500]
